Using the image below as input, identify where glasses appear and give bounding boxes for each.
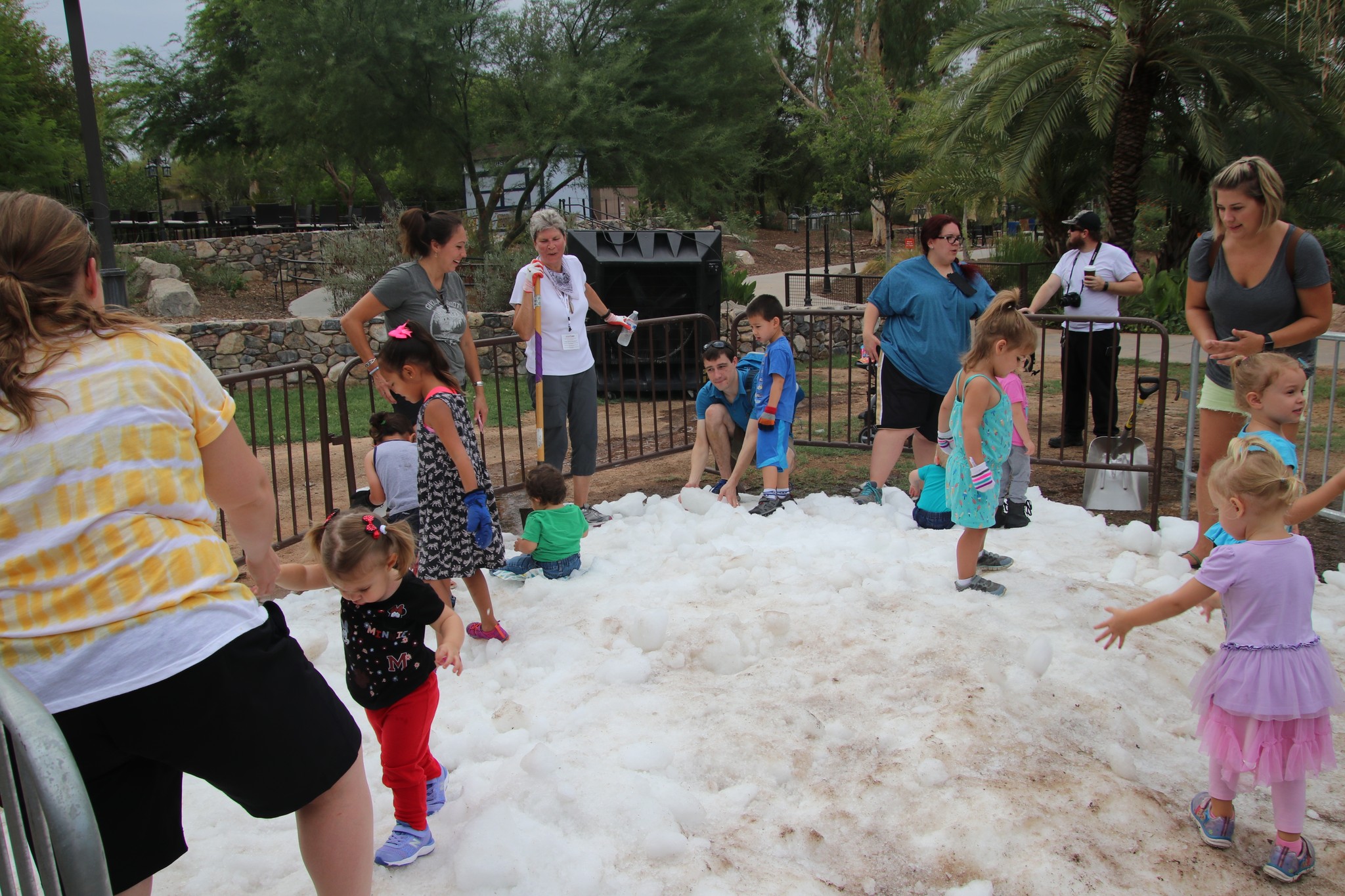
[72,211,92,277]
[701,340,733,354]
[936,236,964,244]
[1069,225,1084,233]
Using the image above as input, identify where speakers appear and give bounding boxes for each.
[567,227,722,400]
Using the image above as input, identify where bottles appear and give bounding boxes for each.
[617,310,639,346]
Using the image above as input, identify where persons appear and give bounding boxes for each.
[339,207,488,427]
[909,288,1035,596]
[1179,156,1333,570]
[364,321,589,643]
[510,210,634,526]
[1094,437,1345,882]
[854,215,995,504]
[275,510,464,865]
[0,190,376,896]
[1204,355,1345,546]
[678,294,796,516]
[1019,209,1144,447]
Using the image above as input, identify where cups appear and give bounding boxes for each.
[1084,265,1096,287]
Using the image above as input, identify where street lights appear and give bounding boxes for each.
[818,205,837,295]
[912,207,926,246]
[787,200,823,307]
[1003,205,1021,236]
[839,205,860,275]
[145,157,172,241]
[72,177,92,224]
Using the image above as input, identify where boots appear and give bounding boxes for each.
[989,504,1007,529]
[1003,498,1032,529]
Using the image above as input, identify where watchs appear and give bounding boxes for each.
[1263,333,1274,352]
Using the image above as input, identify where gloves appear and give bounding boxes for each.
[758,406,777,432]
[937,430,956,455]
[462,487,493,549]
[970,462,996,493]
[523,259,544,293]
[604,313,634,334]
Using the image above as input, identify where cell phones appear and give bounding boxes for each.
[473,400,482,432]
[1218,336,1236,342]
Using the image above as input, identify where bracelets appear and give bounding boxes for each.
[601,309,611,319]
[1102,281,1108,291]
[365,357,377,368]
[369,366,379,375]
[474,381,483,386]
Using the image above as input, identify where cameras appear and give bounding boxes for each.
[1059,292,1080,307]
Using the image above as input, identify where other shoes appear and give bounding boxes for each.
[1048,433,1084,448]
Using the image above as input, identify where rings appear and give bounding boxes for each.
[1236,355,1238,357]
[736,503,739,504]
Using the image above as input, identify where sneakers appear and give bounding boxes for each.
[977,549,1013,571]
[954,574,1006,598]
[466,620,510,643]
[580,503,612,527]
[749,496,797,517]
[1190,792,1236,849]
[709,479,741,501]
[426,763,450,816]
[852,480,882,507]
[374,820,435,867]
[1263,835,1316,883]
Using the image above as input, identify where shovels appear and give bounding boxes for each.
[1081,376,1164,512]
[518,277,545,531]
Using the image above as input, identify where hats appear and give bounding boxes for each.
[1061,210,1101,228]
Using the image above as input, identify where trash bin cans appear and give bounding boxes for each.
[1028,219,1035,231]
[1007,222,1019,236]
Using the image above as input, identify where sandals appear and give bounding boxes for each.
[1178,550,1201,568]
